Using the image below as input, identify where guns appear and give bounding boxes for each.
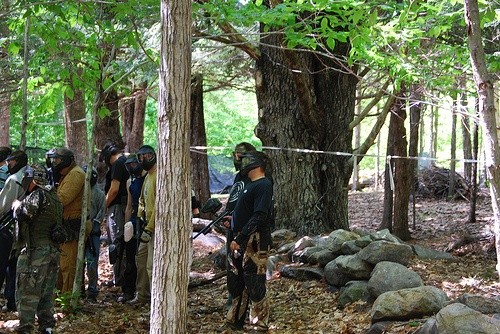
[0,181,143,273]
[193,197,235,240]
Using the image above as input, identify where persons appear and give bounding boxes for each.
[224,141,274,312]
[10,162,64,333]
[0,142,157,314]
[208,151,272,334]
